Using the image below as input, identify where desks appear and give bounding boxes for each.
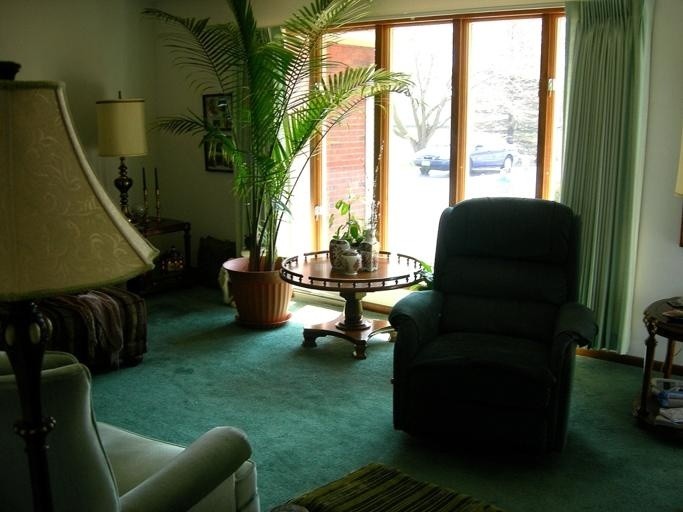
[280,251,430,359]
[632,297,682,446]
[129,216,192,295]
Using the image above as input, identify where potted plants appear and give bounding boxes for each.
[334,193,364,250]
[141,1,417,327]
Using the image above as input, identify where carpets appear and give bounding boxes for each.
[270,461,506,512]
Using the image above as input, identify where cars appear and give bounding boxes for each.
[413,130,520,177]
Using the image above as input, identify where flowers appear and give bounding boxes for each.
[368,199,381,228]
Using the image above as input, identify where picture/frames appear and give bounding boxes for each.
[202,92,234,172]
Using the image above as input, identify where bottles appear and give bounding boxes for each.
[159,244,185,270]
[328,227,380,274]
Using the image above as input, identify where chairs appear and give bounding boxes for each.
[388,197,599,462]
[1,351,259,512]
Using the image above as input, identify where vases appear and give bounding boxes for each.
[339,248,362,276]
[363,230,379,271]
[329,240,350,270]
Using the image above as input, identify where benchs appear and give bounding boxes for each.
[0,287,149,375]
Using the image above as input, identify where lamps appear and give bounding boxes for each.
[96,89,148,214]
[0,78,159,512]
